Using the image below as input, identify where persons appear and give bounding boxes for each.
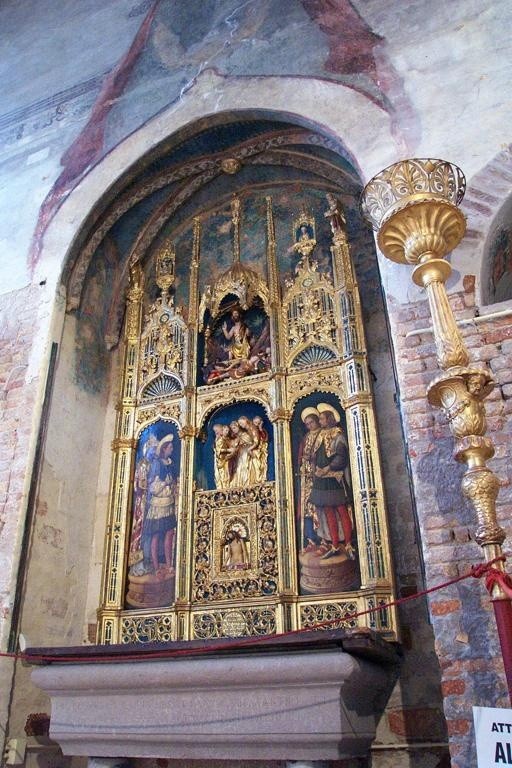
[128,439,160,566]
[322,192,348,234]
[296,224,312,242]
[222,530,249,570]
[296,407,331,557]
[312,402,358,562]
[219,309,255,363]
[204,346,273,389]
[208,413,271,490]
[143,433,177,580]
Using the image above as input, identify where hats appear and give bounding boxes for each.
[317,402,341,424]
[155,433,174,456]
[300,406,320,426]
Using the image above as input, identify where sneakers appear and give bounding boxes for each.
[312,541,330,557]
[320,545,342,560]
[344,543,356,561]
[298,539,316,554]
